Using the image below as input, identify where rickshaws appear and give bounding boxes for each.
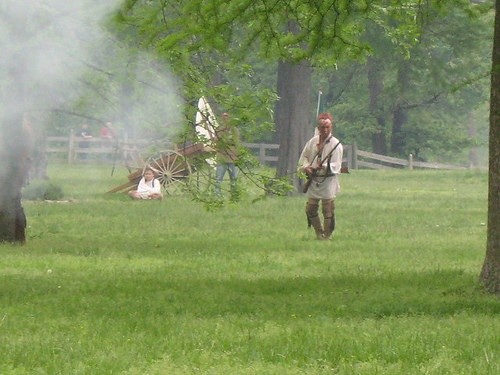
[105,141,217,200]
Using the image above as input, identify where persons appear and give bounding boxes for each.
[78,125,92,160]
[214,113,239,201]
[99,122,113,147]
[297,113,342,239]
[130,170,162,200]
